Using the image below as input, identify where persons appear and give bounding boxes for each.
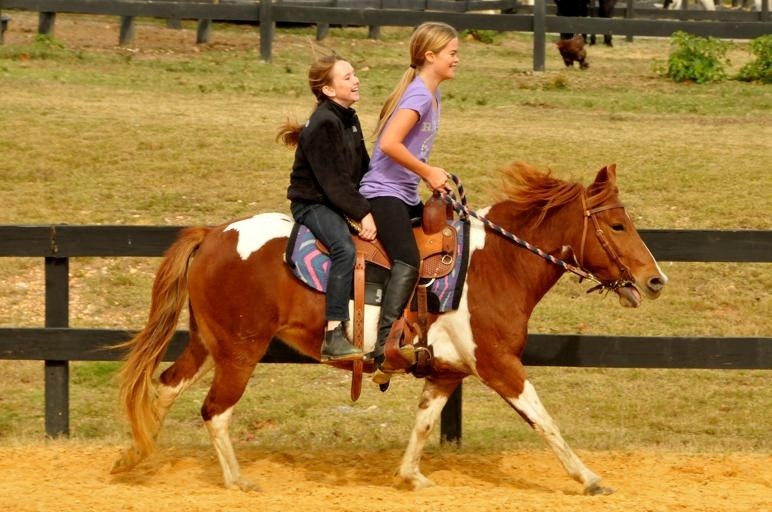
[356,20,465,364]
[285,54,374,363]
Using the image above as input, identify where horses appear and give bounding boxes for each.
[86,159,668,495]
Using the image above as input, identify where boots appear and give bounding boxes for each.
[319,324,363,363]
[372,260,419,385]
